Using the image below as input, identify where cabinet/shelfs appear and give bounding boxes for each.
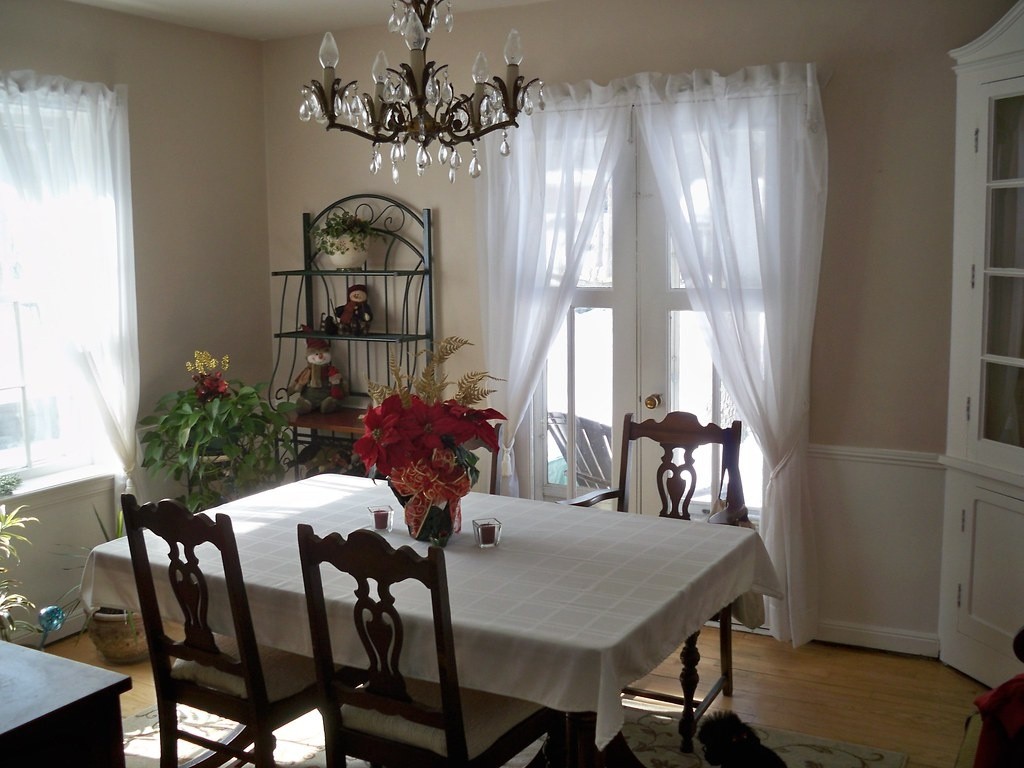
[269,194,433,486]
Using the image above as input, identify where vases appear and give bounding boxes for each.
[401,495,453,541]
[324,234,371,272]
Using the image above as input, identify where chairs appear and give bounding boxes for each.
[298,524,570,768]
[547,414,612,489]
[120,493,360,768]
[554,411,746,754]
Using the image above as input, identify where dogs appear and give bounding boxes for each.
[696,710,787,768]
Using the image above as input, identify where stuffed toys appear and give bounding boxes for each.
[285,442,387,480]
[325,285,374,337]
[287,324,344,416]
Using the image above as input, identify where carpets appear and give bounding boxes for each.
[121,699,909,768]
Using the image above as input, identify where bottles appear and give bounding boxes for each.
[453,499,462,533]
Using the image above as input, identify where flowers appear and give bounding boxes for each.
[352,338,509,489]
[312,213,376,254]
[186,350,233,403]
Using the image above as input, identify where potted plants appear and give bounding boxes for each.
[137,380,299,514]
[46,505,148,665]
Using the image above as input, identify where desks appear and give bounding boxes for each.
[0,639,132,768]
[80,472,786,768]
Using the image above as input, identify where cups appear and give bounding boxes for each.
[367,505,394,532]
[472,518,502,549]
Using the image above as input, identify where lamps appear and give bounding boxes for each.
[300,0,544,183]
